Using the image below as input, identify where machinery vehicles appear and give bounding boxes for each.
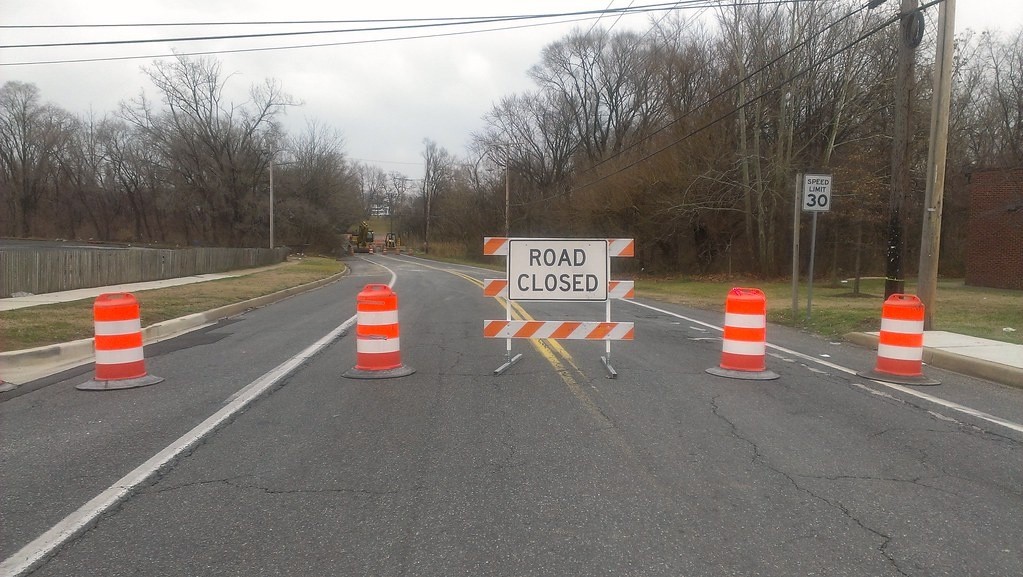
[384,232,397,249]
[351,221,377,253]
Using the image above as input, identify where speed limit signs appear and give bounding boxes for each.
[802,174,832,213]
